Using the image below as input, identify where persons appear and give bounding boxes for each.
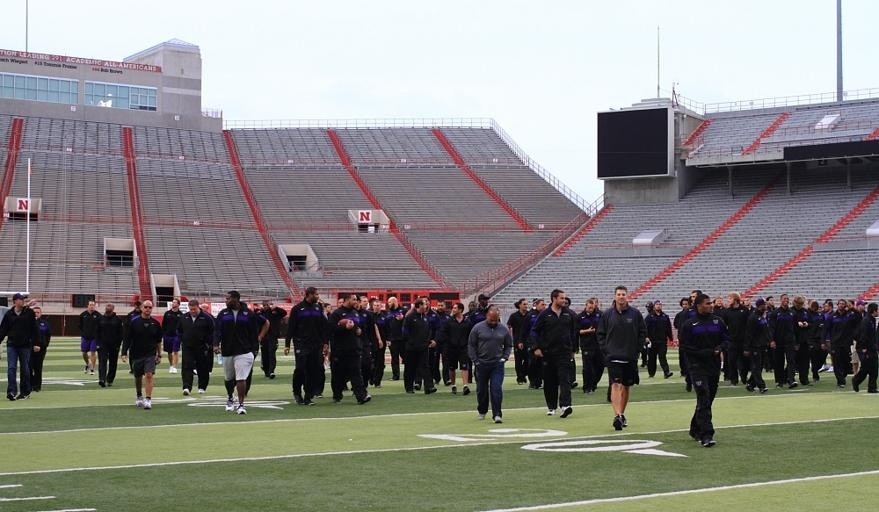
[0,293,37,400]
[29,307,50,392]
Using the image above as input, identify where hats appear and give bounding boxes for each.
[13,293,24,300]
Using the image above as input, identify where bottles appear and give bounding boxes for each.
[216,351,223,364]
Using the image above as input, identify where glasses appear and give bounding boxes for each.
[144,305,152,309]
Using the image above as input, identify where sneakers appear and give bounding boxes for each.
[559,406,574,417]
[514,376,598,395]
[363,371,453,395]
[452,387,457,394]
[136,398,144,408]
[689,429,716,447]
[6,392,31,401]
[226,401,234,411]
[623,417,628,427]
[664,371,675,380]
[463,386,470,394]
[144,399,152,409]
[199,389,206,394]
[547,409,557,416]
[236,404,247,415]
[493,415,502,424]
[613,418,623,430]
[729,377,820,393]
[167,364,278,381]
[83,366,94,375]
[183,388,189,395]
[478,414,486,420]
[852,377,879,394]
[292,389,374,407]
